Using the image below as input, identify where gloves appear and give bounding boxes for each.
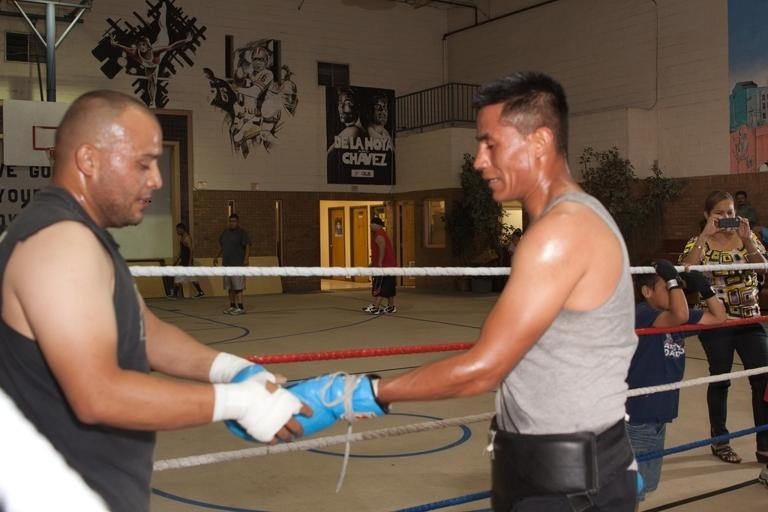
[208,352,265,383]
[211,370,302,443]
[225,363,389,445]
[651,257,681,291]
[682,269,716,298]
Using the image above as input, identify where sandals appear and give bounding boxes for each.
[755,452,768,462]
[711,444,742,463]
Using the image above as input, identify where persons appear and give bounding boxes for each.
[508,229,522,258]
[679,191,768,462]
[360,217,397,315]
[734,191,757,230]
[334,218,342,236]
[199,46,299,159]
[214,214,251,315]
[226,70,642,510]
[168,224,205,301]
[365,92,392,152]
[0,89,313,512]
[108,1,194,109]
[636,256,728,510]
[328,85,368,157]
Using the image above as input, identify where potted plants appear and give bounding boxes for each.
[441,153,516,293]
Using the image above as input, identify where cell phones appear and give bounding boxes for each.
[719,218,740,227]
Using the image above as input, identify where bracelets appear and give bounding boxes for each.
[747,248,760,255]
[691,244,703,249]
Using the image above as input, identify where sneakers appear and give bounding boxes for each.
[228,308,245,316]
[360,304,380,315]
[380,304,397,313]
[223,307,236,314]
[166,295,176,300]
[192,291,205,299]
